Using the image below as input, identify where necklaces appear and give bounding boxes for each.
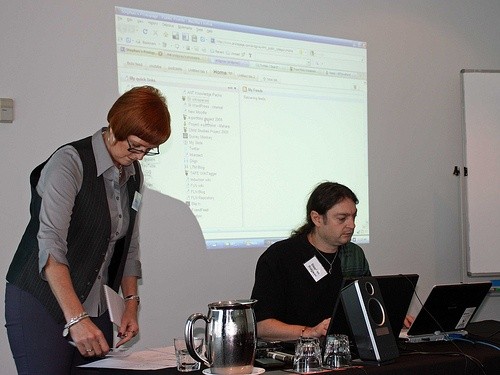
[311,232,339,275]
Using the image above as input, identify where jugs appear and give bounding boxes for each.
[184,299,258,375]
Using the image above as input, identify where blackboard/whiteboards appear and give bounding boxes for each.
[460,71,500,281]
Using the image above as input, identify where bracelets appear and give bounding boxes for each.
[300,326,308,338]
[62,312,90,337]
[124,294,140,305]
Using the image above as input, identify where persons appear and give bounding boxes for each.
[251,181,416,339]
[5,86,172,375]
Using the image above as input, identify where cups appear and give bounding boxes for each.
[322,334,352,370]
[292,337,324,373]
[173,338,203,372]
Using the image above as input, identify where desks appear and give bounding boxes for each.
[73,320,500,375]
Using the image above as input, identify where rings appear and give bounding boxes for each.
[130,330,135,334]
[86,348,94,352]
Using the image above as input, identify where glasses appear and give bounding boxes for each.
[126,139,160,156]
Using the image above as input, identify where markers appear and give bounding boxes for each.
[267,350,294,361]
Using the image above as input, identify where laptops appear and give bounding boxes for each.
[322,273,419,348]
[398,281,492,344]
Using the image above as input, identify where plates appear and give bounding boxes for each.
[202,367,265,375]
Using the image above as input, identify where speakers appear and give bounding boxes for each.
[340,277,398,363]
